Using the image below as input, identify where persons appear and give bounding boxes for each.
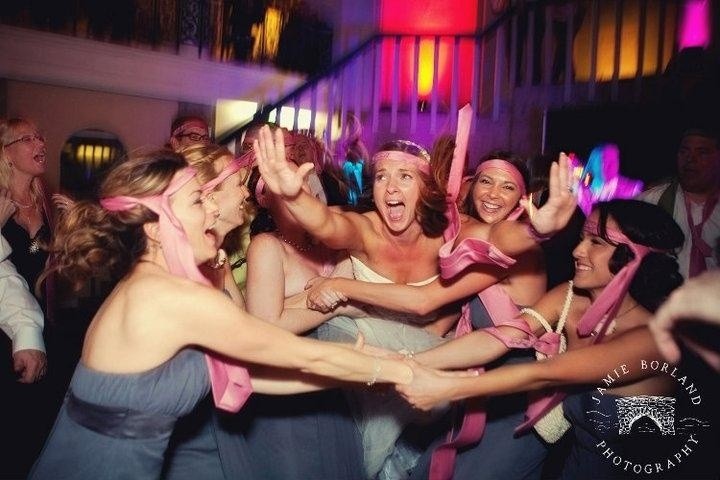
[1,111,719,480]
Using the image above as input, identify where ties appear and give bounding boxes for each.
[684,192,716,278]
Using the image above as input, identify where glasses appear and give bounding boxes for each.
[5,136,47,147]
[178,133,208,141]
